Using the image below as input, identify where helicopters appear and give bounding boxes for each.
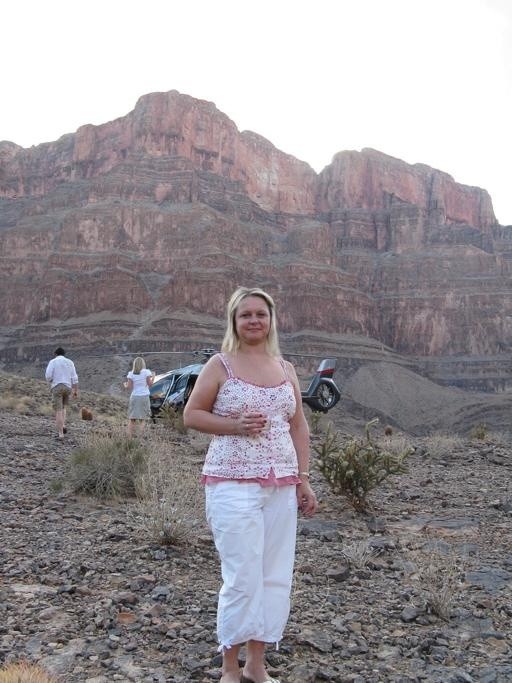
[86,347,341,424]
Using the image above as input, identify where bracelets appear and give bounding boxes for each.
[300,471,311,479]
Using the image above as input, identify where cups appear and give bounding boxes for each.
[257,411,273,431]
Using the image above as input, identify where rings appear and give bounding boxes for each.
[245,423,249,428]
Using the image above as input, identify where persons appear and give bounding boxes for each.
[123,357,156,435]
[179,285,321,683]
[44,346,80,439]
